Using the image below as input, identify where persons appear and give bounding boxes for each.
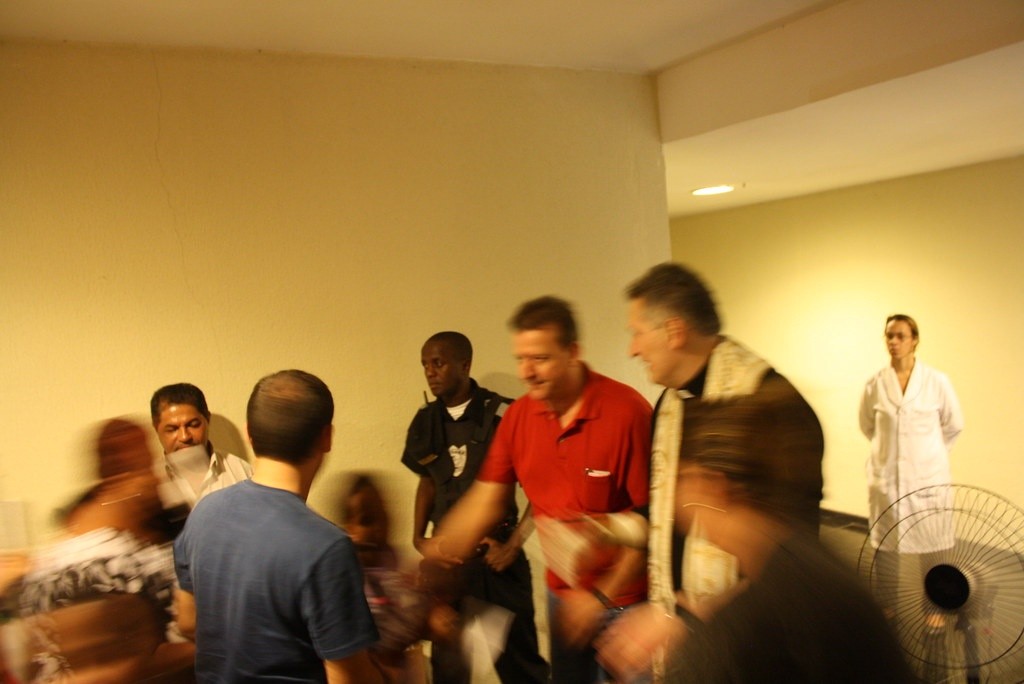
[333,471,460,684]
[400,331,536,684]
[595,395,917,684]
[174,369,397,684]
[577,262,824,684]
[419,295,652,684]
[0,382,254,684]
[859,315,963,684]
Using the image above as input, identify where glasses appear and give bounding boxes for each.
[885,330,914,341]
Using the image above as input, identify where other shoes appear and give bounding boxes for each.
[923,611,948,637]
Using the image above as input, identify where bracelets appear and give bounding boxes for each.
[589,585,614,610]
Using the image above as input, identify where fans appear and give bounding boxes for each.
[856,484,1024,684]
